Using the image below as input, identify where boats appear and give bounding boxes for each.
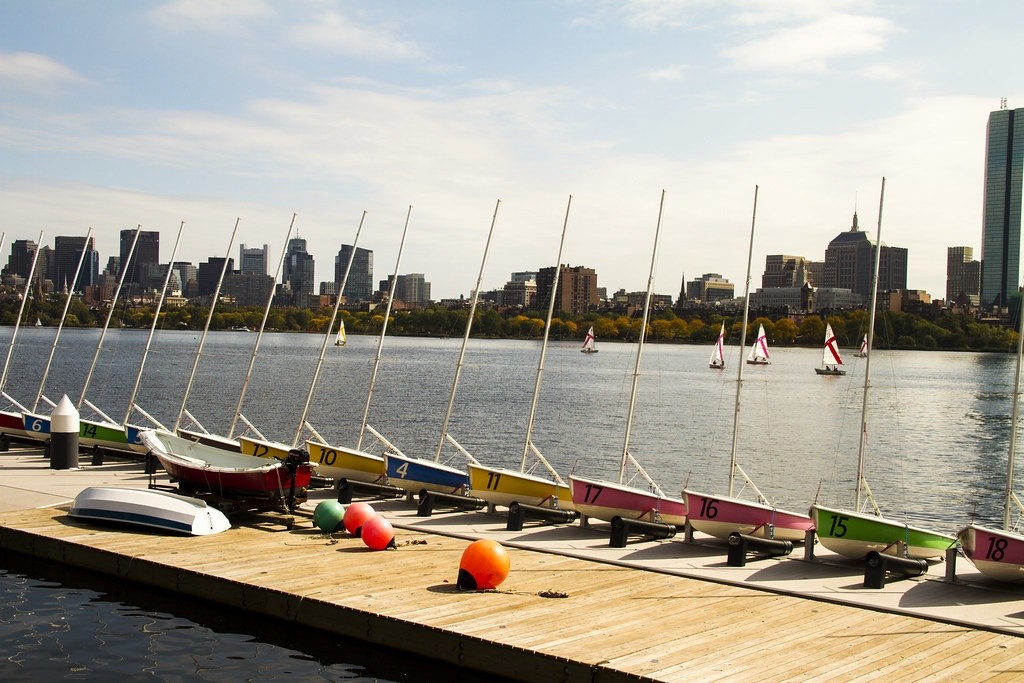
[138,423,311,505]
[69,485,233,535]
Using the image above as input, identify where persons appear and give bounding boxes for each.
[713,359,725,366]
[754,357,766,361]
[826,364,839,371]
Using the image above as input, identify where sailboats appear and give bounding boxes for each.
[681,182,817,544]
[579,325,598,354]
[2,208,369,459]
[811,176,960,561]
[333,314,348,346]
[565,188,688,527]
[745,323,772,365]
[304,198,413,491]
[383,198,488,504]
[464,195,578,516]
[852,332,868,357]
[708,319,726,370]
[956,275,1024,587]
[814,322,848,377]
